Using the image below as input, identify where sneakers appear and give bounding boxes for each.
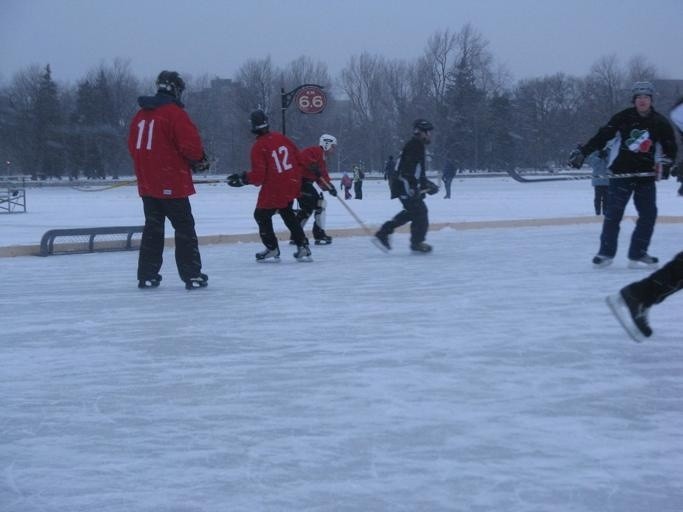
[375,231,392,251]
[289,235,332,259]
[411,241,433,253]
[255,246,281,260]
[140,272,162,282]
[619,282,653,338]
[592,253,614,264]
[627,252,658,264]
[181,273,209,284]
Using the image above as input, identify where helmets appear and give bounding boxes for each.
[413,119,434,136]
[156,68,185,101]
[629,80,656,104]
[319,133,337,151]
[249,109,270,134]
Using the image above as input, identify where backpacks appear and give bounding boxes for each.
[358,169,364,179]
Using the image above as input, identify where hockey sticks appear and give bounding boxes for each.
[504,169,658,184]
[73,179,137,192]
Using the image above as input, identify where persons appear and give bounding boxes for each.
[588,149,609,215]
[352,165,362,199]
[568,83,677,264]
[384,155,396,185]
[442,159,458,199]
[290,134,338,242]
[128,71,209,290]
[227,110,311,259]
[340,171,353,200]
[374,120,439,252]
[619,95,683,337]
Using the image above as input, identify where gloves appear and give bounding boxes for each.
[568,148,585,170]
[424,181,439,195]
[189,153,210,176]
[226,170,249,188]
[654,159,673,181]
[312,163,321,178]
[325,182,337,197]
